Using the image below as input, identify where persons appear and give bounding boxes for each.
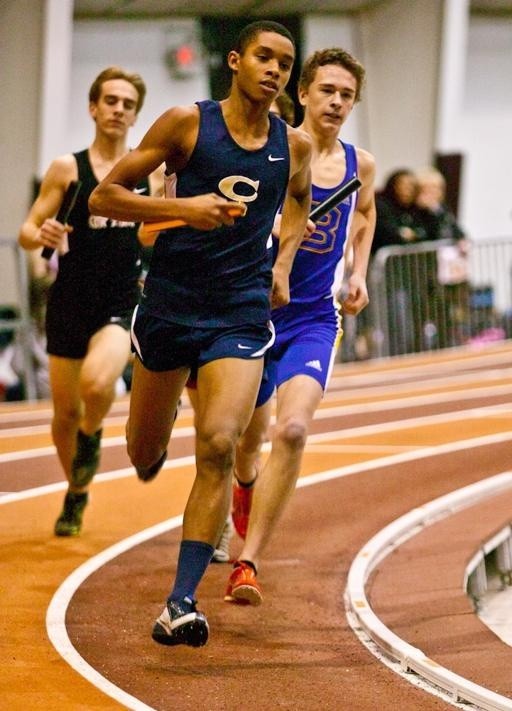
[24,165,470,401]
[17,66,167,537]
[223,47,377,606]
[88,20,313,648]
[169,86,298,563]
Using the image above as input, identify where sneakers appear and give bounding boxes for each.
[134,409,177,482]
[69,428,103,488]
[54,490,88,537]
[209,514,236,563]
[232,466,260,541]
[223,559,263,607]
[151,598,210,648]
